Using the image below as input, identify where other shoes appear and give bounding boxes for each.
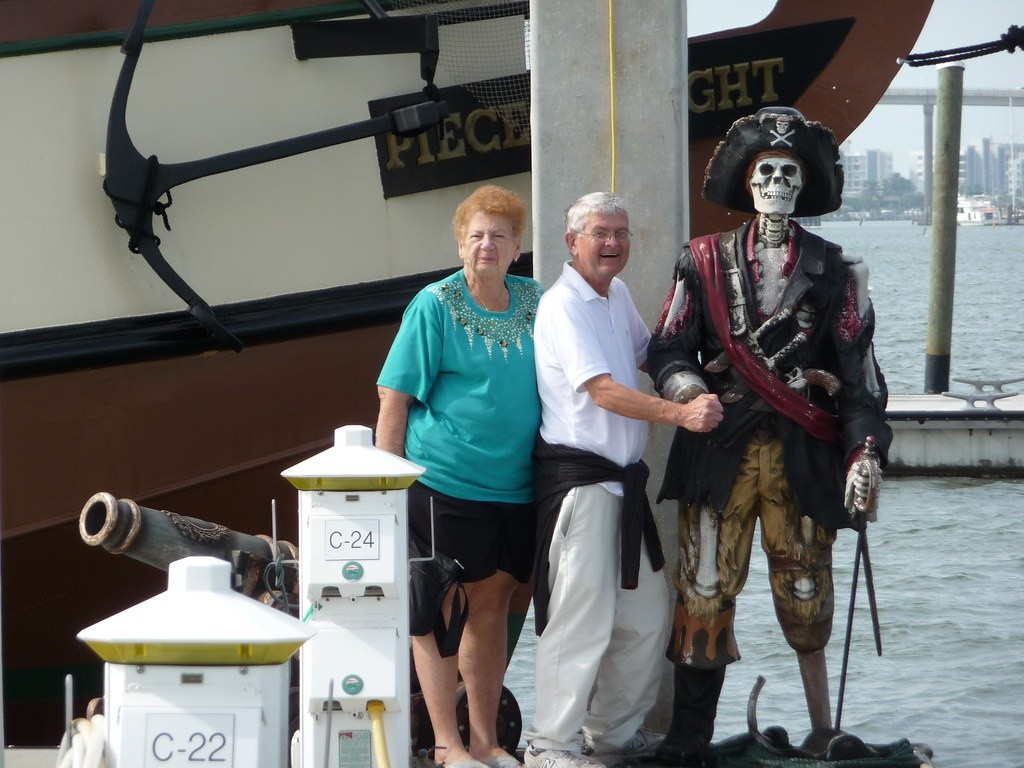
[488,755,522,768]
[434,760,488,768]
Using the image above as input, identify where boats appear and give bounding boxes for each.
[0,0,937,757]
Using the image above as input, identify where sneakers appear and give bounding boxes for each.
[615,727,668,757]
[523,743,608,768]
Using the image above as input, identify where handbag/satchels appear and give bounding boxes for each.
[407,528,463,637]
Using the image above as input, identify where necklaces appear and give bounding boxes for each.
[466,281,505,311]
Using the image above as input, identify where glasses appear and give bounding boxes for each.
[574,228,634,242]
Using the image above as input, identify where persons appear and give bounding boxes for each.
[373,184,546,768]
[524,191,724,768]
[646,104,894,765]
